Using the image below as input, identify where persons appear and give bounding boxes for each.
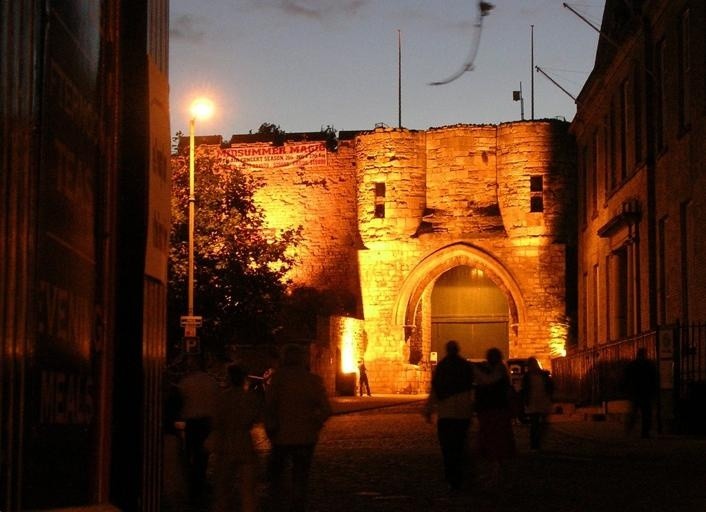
[622,347,660,439]
[473,345,516,468]
[519,356,549,453]
[160,338,332,510]
[358,359,372,397]
[421,342,506,484]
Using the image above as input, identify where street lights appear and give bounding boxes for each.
[182,91,218,348]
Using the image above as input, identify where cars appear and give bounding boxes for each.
[504,356,555,399]
